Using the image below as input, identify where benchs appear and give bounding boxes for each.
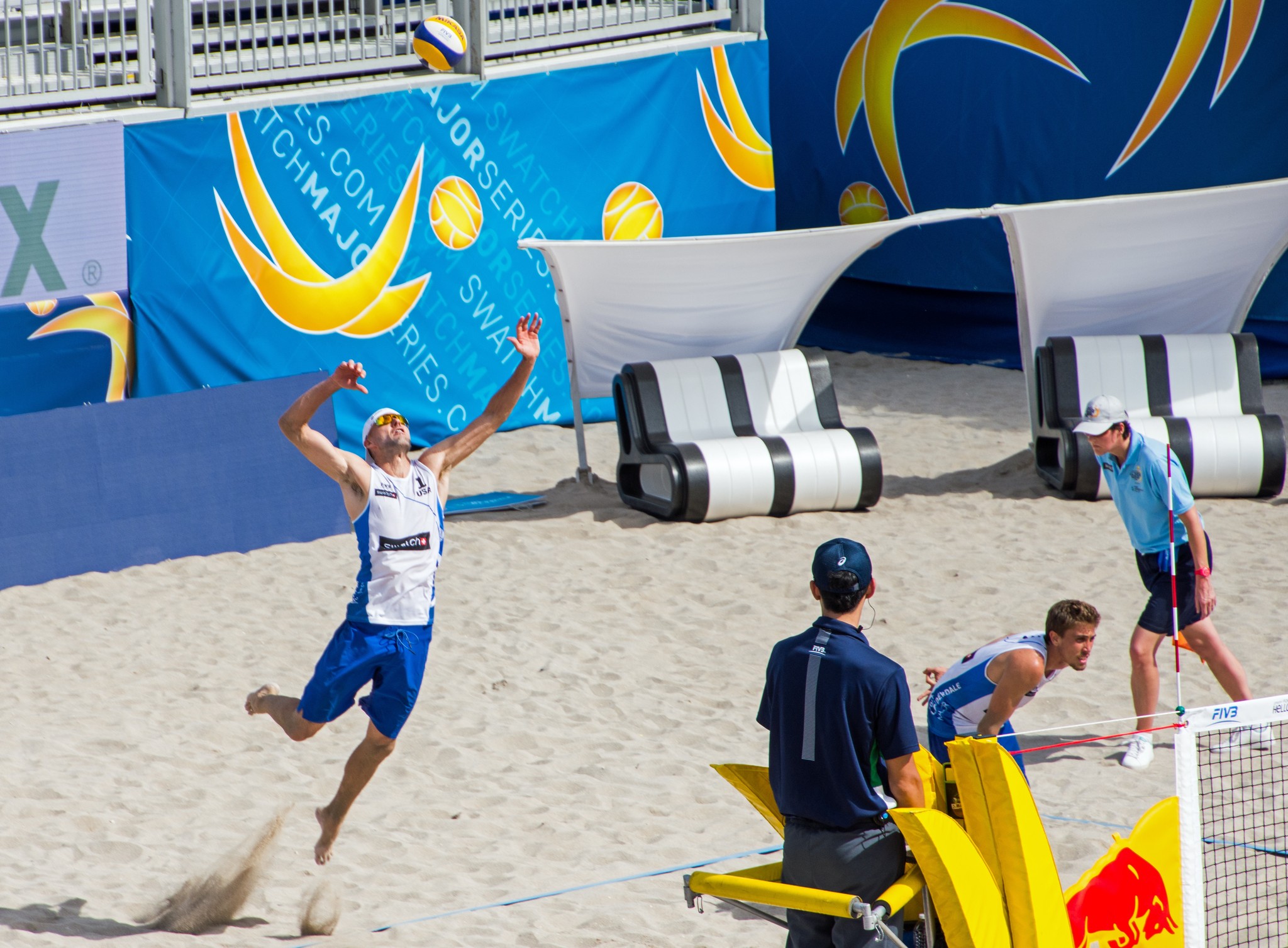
[613,347,882,523]
[0,0,675,93]
[1036,333,1287,499]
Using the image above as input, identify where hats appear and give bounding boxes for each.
[362,408,401,466]
[811,537,872,594]
[1072,394,1129,435]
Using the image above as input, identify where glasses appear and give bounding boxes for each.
[372,414,410,429]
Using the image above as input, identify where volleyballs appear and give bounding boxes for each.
[412,14,467,70]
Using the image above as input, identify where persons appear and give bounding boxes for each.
[756,536,927,948]
[917,598,1101,783]
[245,311,543,866]
[1073,395,1277,770]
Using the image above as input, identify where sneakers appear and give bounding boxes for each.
[1122,733,1154,770]
[1210,723,1276,754]
